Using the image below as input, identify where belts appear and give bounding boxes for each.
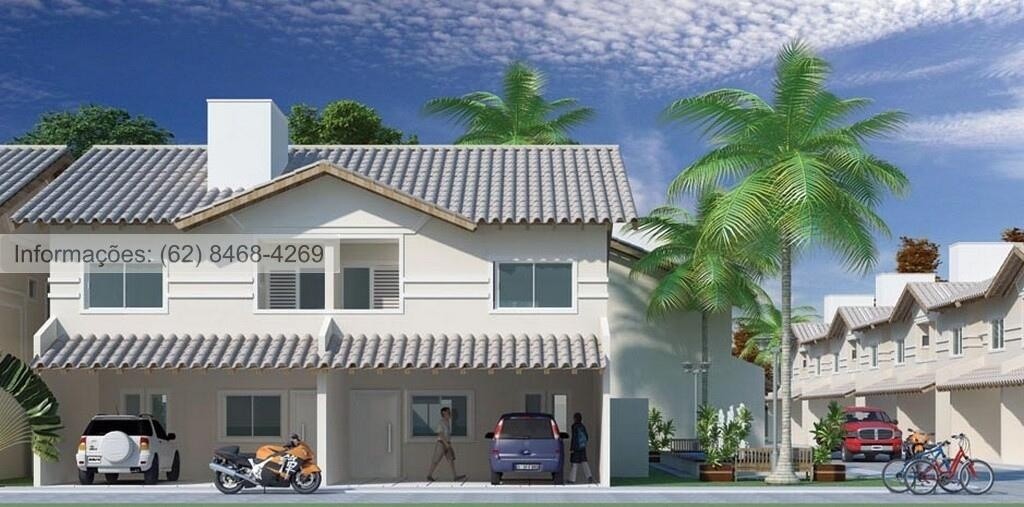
[438,439,452,446]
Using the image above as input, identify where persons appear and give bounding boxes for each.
[563,411,594,484]
[421,404,466,482]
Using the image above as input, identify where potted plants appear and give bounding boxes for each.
[648,407,677,464]
[808,400,850,482]
[690,402,755,483]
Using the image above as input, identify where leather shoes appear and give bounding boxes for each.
[455,474,466,481]
[428,475,435,482]
